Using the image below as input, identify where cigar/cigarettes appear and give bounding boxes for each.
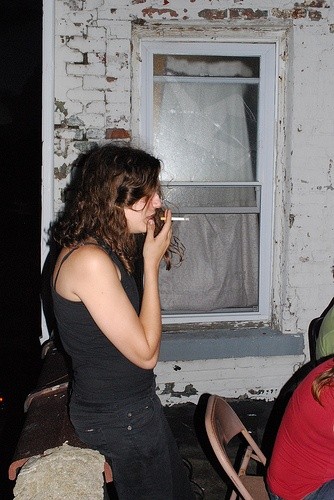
[161,216,189,221]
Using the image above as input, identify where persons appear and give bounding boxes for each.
[47,142,186,500]
[315,303,334,363]
[265,356,334,500]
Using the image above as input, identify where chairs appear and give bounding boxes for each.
[204,394,269,500]
